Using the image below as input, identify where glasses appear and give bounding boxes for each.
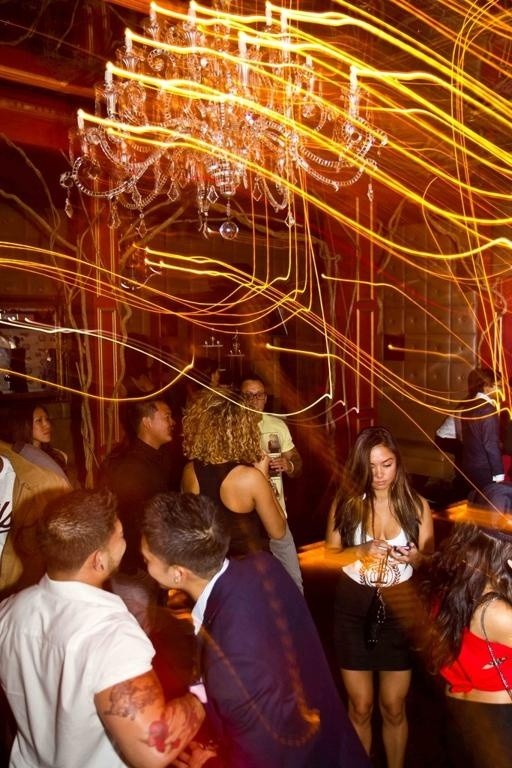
[243,392,266,400]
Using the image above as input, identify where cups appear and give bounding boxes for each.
[267,453,281,480]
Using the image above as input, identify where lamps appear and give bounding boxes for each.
[59,1,390,240]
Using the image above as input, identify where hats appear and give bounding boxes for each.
[474,484,512,542]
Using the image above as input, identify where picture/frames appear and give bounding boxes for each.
[1,300,64,401]
